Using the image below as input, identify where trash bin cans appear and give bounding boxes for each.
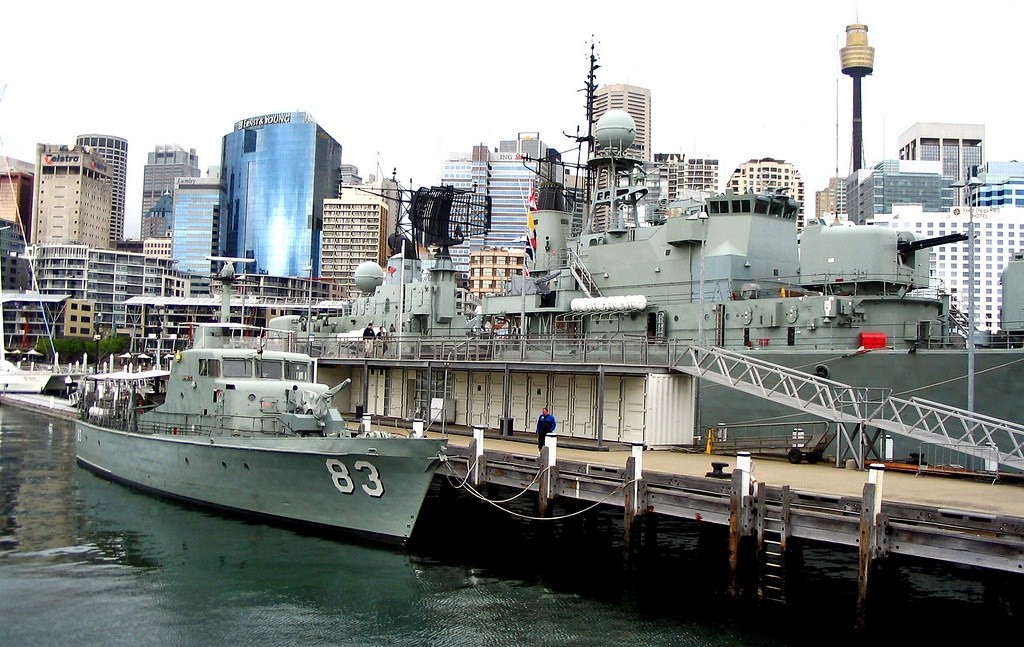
[499,418,514,436]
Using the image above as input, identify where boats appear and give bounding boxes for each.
[74,318,450,549]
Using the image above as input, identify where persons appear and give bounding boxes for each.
[363,323,375,358]
[390,324,396,332]
[375,326,388,355]
[536,408,556,452]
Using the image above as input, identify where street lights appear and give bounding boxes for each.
[947,176,984,438]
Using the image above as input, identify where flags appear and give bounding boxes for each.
[525,186,539,261]
